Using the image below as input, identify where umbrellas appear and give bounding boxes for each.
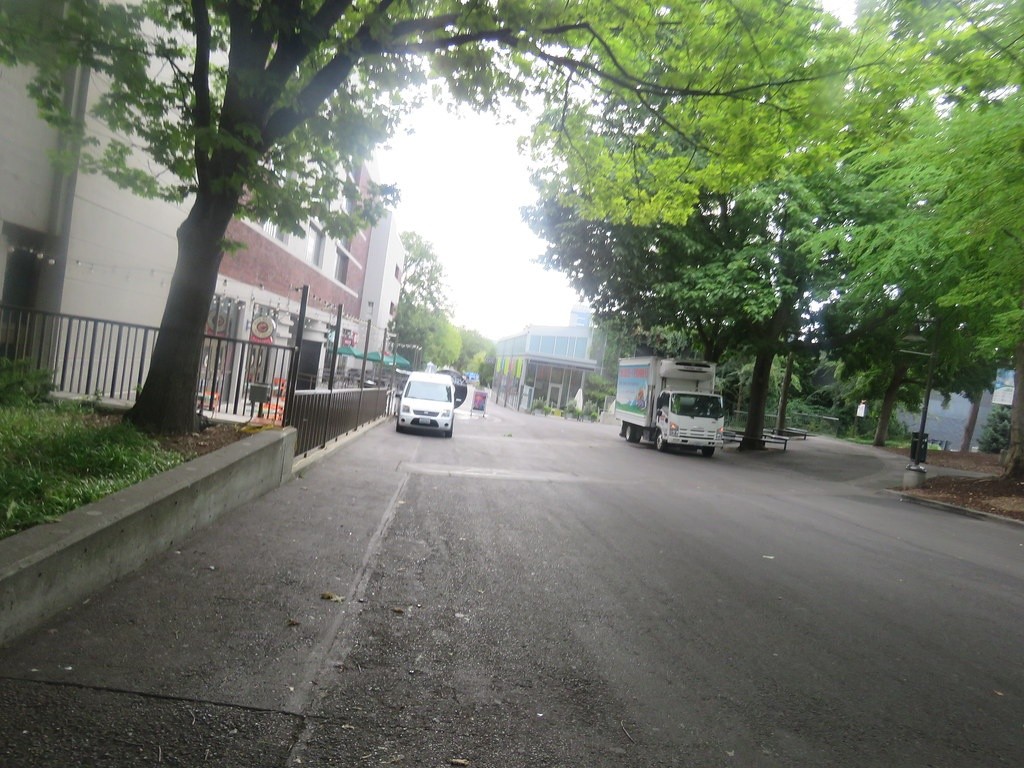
[328,345,410,368]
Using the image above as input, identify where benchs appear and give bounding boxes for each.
[723,426,808,452]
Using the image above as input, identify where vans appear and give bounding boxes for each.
[397,371,458,439]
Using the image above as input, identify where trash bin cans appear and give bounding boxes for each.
[911,432,929,463]
[364,380,376,388]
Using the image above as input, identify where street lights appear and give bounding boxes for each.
[901,317,942,489]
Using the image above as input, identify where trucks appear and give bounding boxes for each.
[613,354,726,459]
